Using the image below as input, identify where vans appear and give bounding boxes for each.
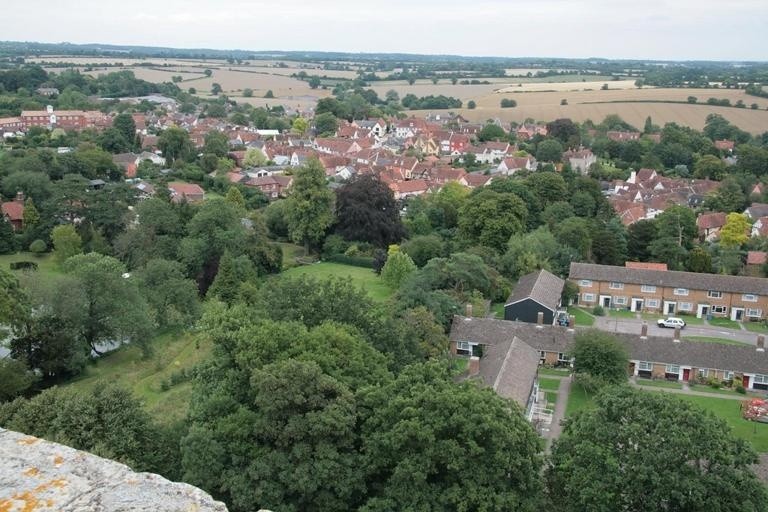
[656,316,686,329]
